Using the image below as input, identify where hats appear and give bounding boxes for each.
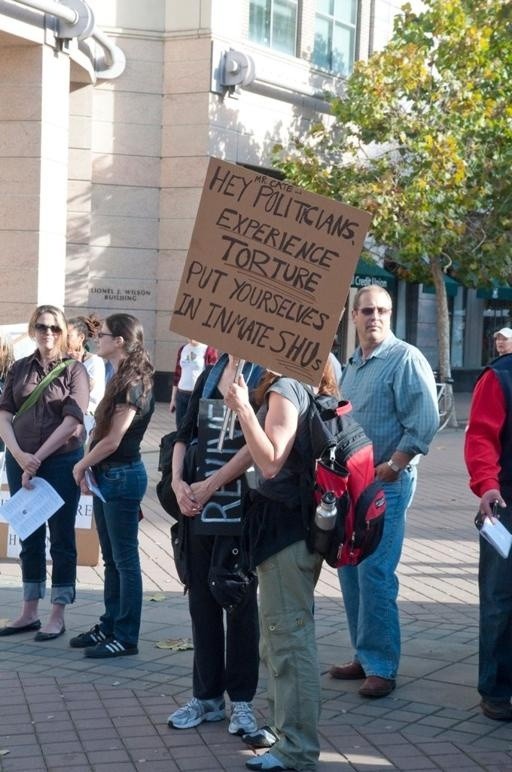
[494,327,511,339]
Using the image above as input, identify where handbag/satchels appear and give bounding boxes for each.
[156,430,207,528]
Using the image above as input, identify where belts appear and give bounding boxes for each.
[104,455,141,462]
[178,388,193,396]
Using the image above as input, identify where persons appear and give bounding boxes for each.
[332,285,440,696]
[225,356,338,711]
[0,304,86,641]
[0,338,13,477]
[168,337,218,429]
[494,326,511,359]
[71,315,153,658]
[465,353,511,721]
[168,350,261,734]
[65,312,107,433]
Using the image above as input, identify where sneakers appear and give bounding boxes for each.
[245,749,315,771]
[241,725,281,747]
[70,624,111,648]
[85,634,138,660]
[228,699,259,737]
[167,696,226,730]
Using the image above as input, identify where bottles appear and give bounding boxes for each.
[312,493,340,559]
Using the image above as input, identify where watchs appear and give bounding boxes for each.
[387,458,401,473]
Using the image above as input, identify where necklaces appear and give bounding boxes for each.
[233,360,242,368]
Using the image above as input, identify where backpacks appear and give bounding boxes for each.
[294,381,386,568]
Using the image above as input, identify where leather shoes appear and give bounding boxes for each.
[329,661,366,680]
[1,620,41,636]
[481,697,512,723]
[35,621,65,642]
[359,675,396,698]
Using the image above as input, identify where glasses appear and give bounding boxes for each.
[355,307,393,316]
[34,323,61,334]
[98,332,113,338]
